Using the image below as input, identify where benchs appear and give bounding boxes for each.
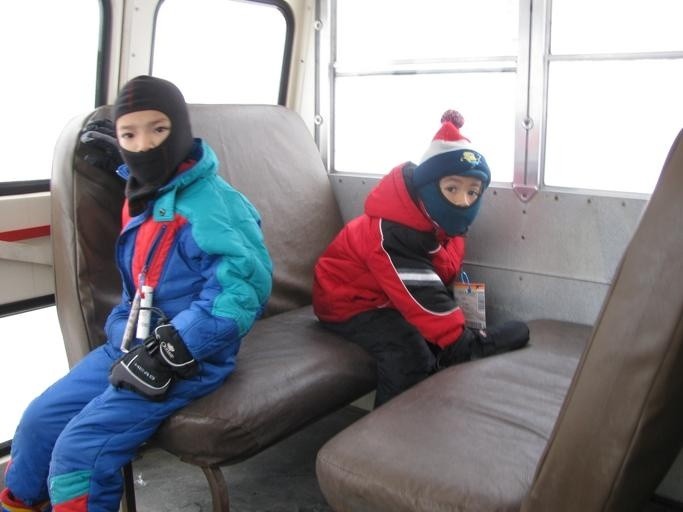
[46,99,391,512]
[310,128,682,512]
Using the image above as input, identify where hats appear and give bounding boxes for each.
[411,109,493,235]
[112,75,195,188]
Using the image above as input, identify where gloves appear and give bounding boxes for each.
[108,323,198,402]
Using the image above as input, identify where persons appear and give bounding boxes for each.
[310,108,533,414]
[0,74,273,512]
